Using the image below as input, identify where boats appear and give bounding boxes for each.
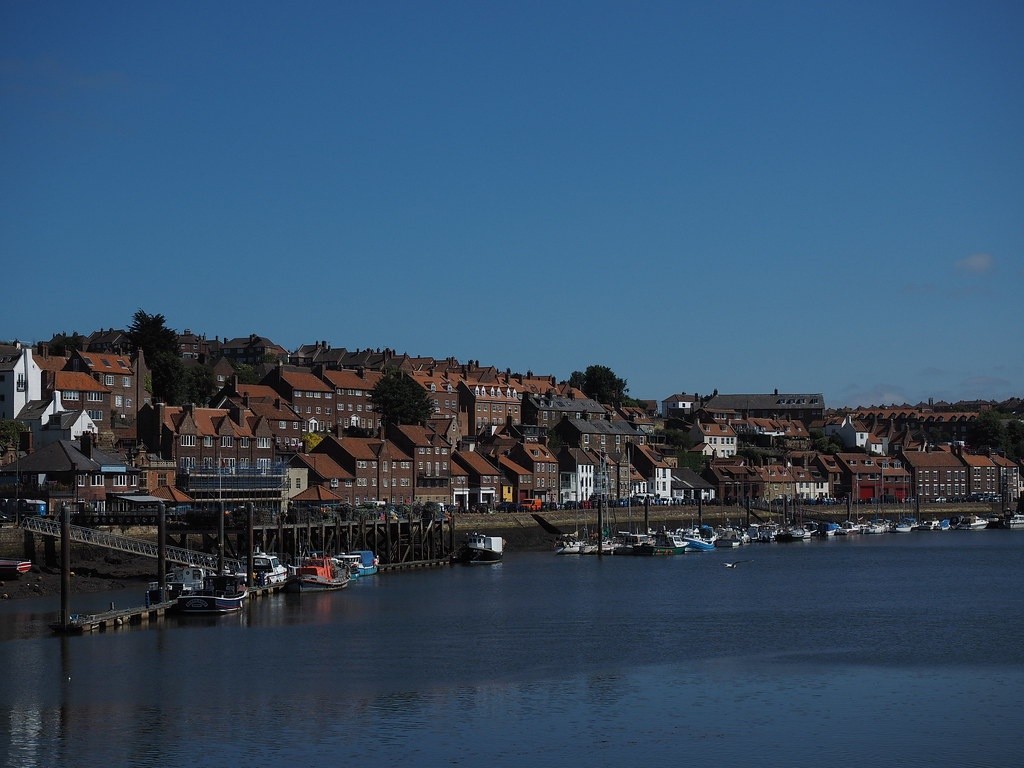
[0,558,33,582]
[252,550,379,592]
[464,531,506,565]
[147,566,218,599]
[173,574,248,614]
[553,516,1023,555]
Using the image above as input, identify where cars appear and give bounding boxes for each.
[541,493,915,511]
[931,496,947,503]
[949,494,1002,502]
[496,501,520,513]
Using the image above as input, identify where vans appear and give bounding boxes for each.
[519,499,542,512]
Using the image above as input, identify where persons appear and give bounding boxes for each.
[444,504,452,525]
[252,568,266,587]
